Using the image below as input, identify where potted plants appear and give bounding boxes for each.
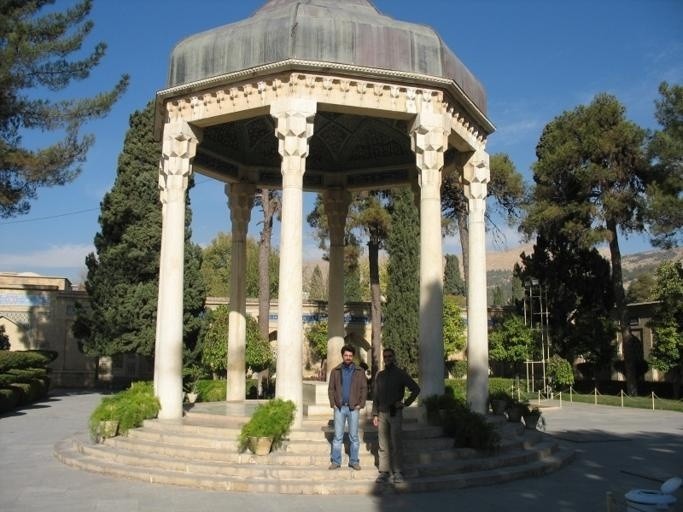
[235,397,298,455]
[422,388,547,455]
[88,381,162,440]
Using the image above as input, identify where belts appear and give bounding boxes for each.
[342,402,349,405]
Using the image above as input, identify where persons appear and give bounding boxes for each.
[328,344,368,470]
[372,348,420,484]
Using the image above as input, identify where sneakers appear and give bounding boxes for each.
[393,473,400,483]
[328,464,341,470]
[376,473,388,484]
[352,463,360,470]
